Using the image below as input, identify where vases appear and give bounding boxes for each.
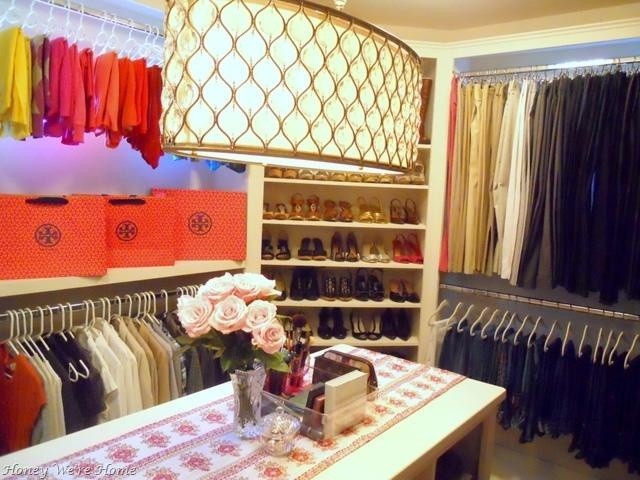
[225,358,266,440]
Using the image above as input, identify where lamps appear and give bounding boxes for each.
[157,0,426,178]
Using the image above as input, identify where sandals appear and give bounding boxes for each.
[264,268,420,302]
[262,231,423,263]
[267,167,412,184]
[264,193,420,224]
[411,161,425,184]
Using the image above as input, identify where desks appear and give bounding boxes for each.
[0,344,508,480]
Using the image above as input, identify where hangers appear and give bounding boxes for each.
[0,0,170,71]
[451,53,640,86]
[428,283,640,369]
[1,280,204,383]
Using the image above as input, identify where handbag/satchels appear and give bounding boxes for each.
[151,188,247,260]
[0,193,108,280]
[71,194,175,268]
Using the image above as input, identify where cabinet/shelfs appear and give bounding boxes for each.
[0,0,258,451]
[248,39,440,369]
[428,1,640,480]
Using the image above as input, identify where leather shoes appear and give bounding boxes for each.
[285,308,409,341]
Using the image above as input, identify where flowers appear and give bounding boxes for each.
[175,269,296,377]
